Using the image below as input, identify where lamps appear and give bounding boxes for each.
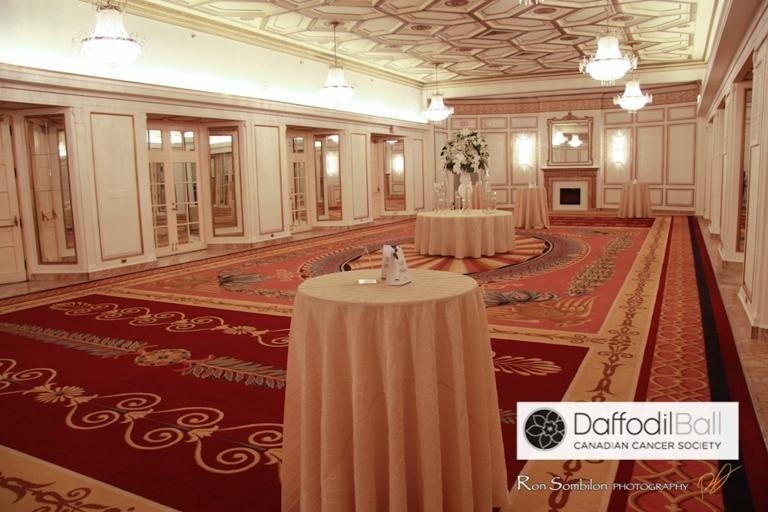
[320,23,352,91]
[553,130,583,149]
[75,3,142,57]
[425,61,452,122]
[577,0,653,114]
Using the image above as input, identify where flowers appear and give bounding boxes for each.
[437,128,492,178]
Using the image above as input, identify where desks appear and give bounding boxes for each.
[515,186,550,230]
[619,183,653,220]
[289,268,511,512]
[410,207,514,260]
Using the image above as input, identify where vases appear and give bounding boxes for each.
[458,170,473,209]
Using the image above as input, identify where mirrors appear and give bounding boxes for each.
[546,117,594,166]
